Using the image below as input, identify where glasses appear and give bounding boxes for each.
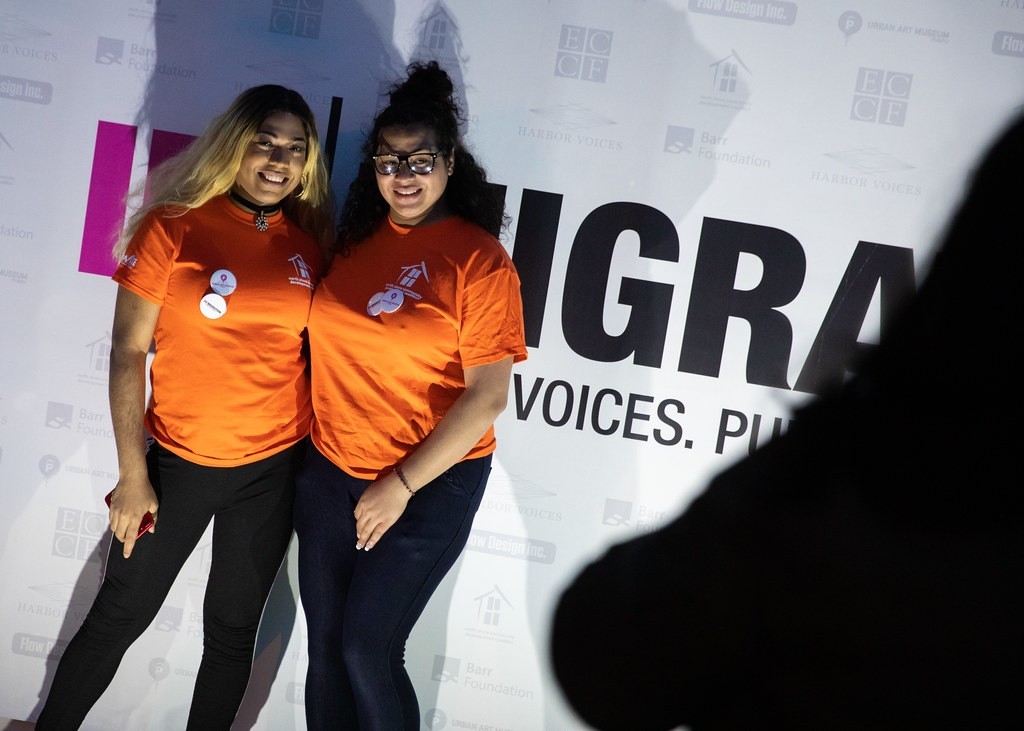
[372,148,442,176]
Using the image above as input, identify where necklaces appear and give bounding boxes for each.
[393,467,415,496]
[230,188,282,232]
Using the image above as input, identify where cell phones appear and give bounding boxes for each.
[104,490,155,538]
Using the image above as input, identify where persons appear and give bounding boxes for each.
[33,84,335,731]
[298,59,529,731]
